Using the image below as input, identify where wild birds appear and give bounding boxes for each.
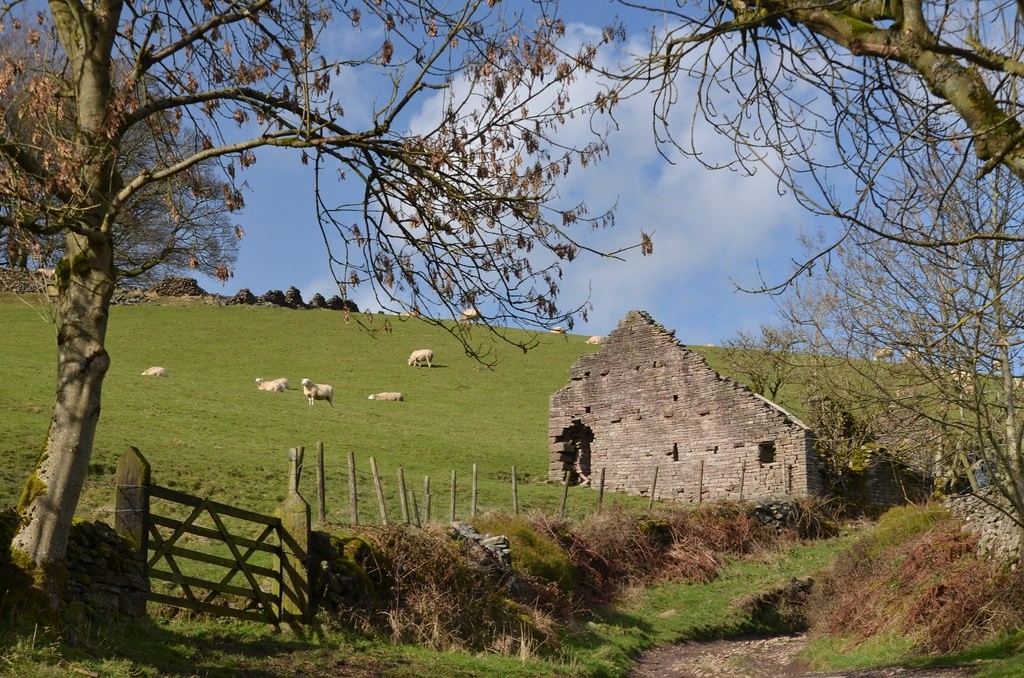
[550,326,564,334]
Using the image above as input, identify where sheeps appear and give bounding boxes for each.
[407,349,433,368]
[141,366,169,377]
[300,378,334,408]
[458,308,481,325]
[401,304,420,316]
[368,392,403,401]
[255,377,289,392]
[871,344,1024,397]
[583,336,604,345]
